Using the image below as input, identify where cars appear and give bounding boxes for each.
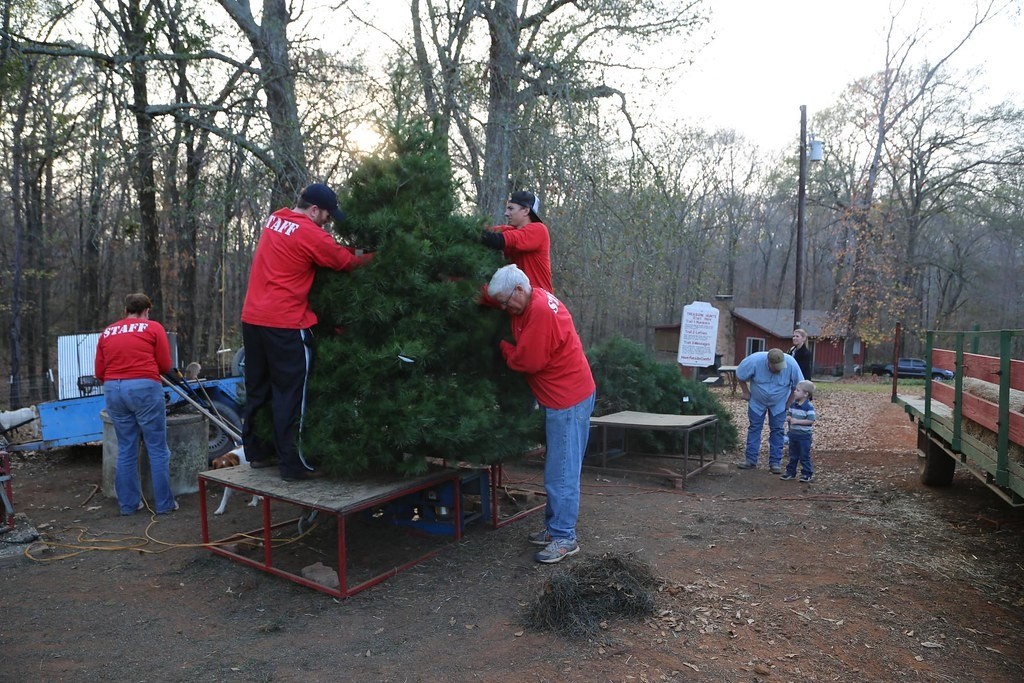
[872,358,954,381]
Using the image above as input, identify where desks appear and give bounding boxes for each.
[196,410,720,599]
[718,365,738,392]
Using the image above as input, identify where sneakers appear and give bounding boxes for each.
[799,474,813,482]
[737,459,756,469]
[780,473,797,480]
[770,464,781,473]
[527,528,551,545]
[248,455,284,468]
[281,463,326,481]
[535,538,580,563]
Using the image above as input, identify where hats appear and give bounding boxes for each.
[301,184,347,220]
[509,190,544,223]
[767,348,787,371]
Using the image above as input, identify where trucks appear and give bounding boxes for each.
[35,341,247,465]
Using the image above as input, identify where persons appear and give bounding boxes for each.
[735,348,804,475]
[240,184,374,482]
[781,380,815,482]
[477,192,555,297]
[95,293,179,515]
[441,264,597,563]
[783,329,812,444]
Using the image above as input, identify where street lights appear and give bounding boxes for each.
[794,140,822,331]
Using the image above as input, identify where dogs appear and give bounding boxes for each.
[212,445,265,515]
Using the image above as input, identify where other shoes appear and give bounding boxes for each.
[137,501,144,510]
[173,500,180,510]
[783,434,792,444]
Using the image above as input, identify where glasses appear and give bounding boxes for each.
[498,284,520,305]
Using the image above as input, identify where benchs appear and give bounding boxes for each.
[702,376,720,384]
